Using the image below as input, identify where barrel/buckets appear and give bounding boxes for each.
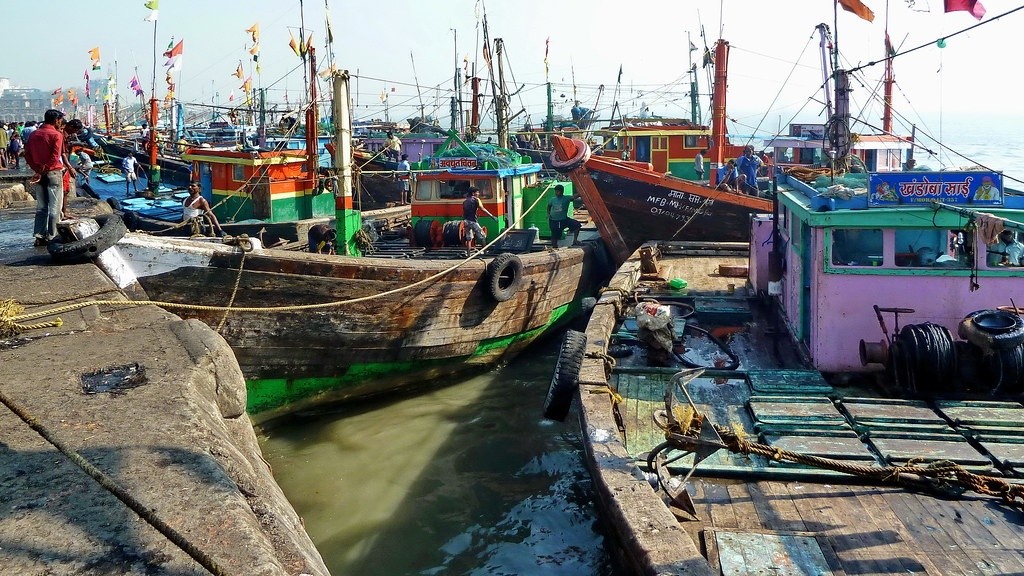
[670,278,687,288]
[727,284,736,293]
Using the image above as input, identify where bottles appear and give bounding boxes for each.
[527,224,540,241]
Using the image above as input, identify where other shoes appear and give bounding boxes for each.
[33,240,47,246]
[405,202,410,205]
[126,192,131,195]
[63,216,79,220]
[11,166,19,169]
[134,189,139,192]
[48,236,60,243]
[572,241,584,246]
[401,202,405,205]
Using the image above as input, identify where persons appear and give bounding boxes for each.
[140,124,150,154]
[716,145,770,198]
[122,151,141,197]
[308,224,337,255]
[546,185,585,247]
[177,135,196,155]
[694,149,707,180]
[486,137,492,144]
[381,132,402,162]
[398,154,412,206]
[986,228,1024,267]
[462,186,498,257]
[178,181,227,238]
[622,145,630,161]
[520,124,541,149]
[0,109,94,244]
[973,176,1000,202]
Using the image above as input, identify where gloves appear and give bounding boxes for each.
[219,230,227,236]
[491,215,499,223]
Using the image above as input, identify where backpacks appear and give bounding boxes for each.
[22,129,31,140]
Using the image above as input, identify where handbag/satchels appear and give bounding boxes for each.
[9,141,19,154]
[61,169,77,201]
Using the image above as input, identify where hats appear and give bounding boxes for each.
[554,184,564,193]
[729,159,738,166]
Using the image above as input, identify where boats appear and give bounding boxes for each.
[539,17,1024,576]
[73,11,917,374]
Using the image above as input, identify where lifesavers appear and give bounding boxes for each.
[549,139,592,173]
[539,328,588,423]
[486,252,524,302]
[579,296,597,327]
[123,211,140,229]
[106,197,121,211]
[962,308,1024,349]
[46,214,128,264]
